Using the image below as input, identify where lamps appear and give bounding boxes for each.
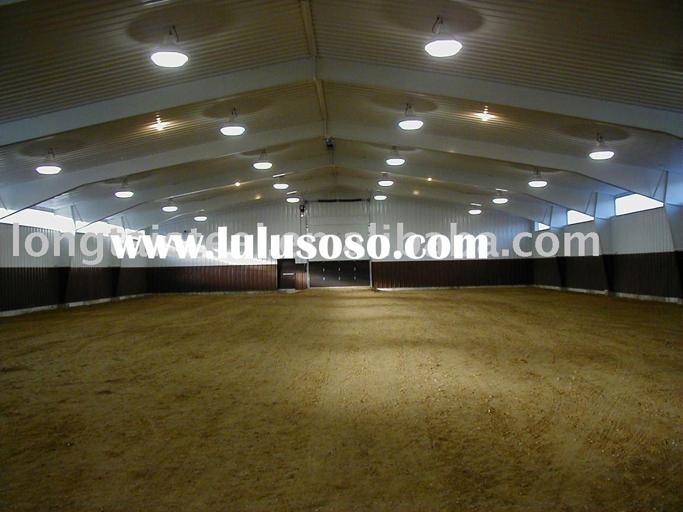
[28,27,289,199]
[375,25,616,192]
